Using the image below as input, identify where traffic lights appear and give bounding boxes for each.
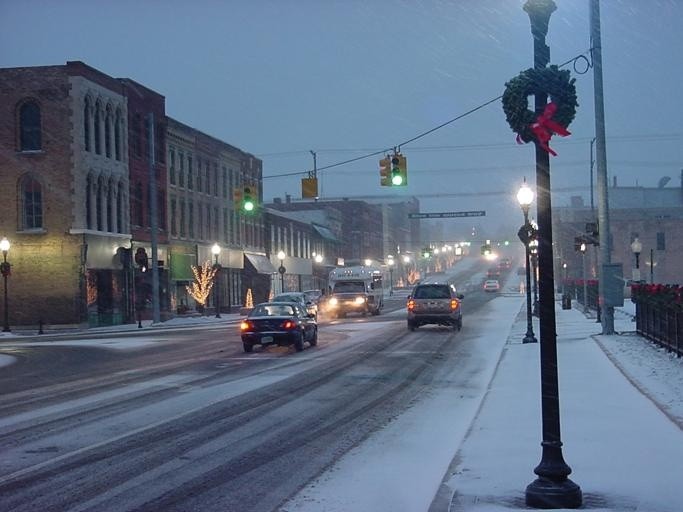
[575,241,586,255]
[240,184,254,214]
[230,185,242,216]
[378,155,391,189]
[389,154,408,189]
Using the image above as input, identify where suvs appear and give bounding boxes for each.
[403,280,465,332]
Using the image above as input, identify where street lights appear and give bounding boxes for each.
[387,237,470,295]
[314,254,323,290]
[211,242,225,319]
[0,238,13,332]
[526,218,541,316]
[276,248,287,293]
[630,236,642,284]
[515,176,538,347]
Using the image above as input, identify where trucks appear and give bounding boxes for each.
[323,265,384,317]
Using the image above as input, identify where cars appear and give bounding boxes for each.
[481,279,500,291]
[310,287,323,308]
[237,299,319,353]
[483,250,513,277]
[277,292,318,321]
